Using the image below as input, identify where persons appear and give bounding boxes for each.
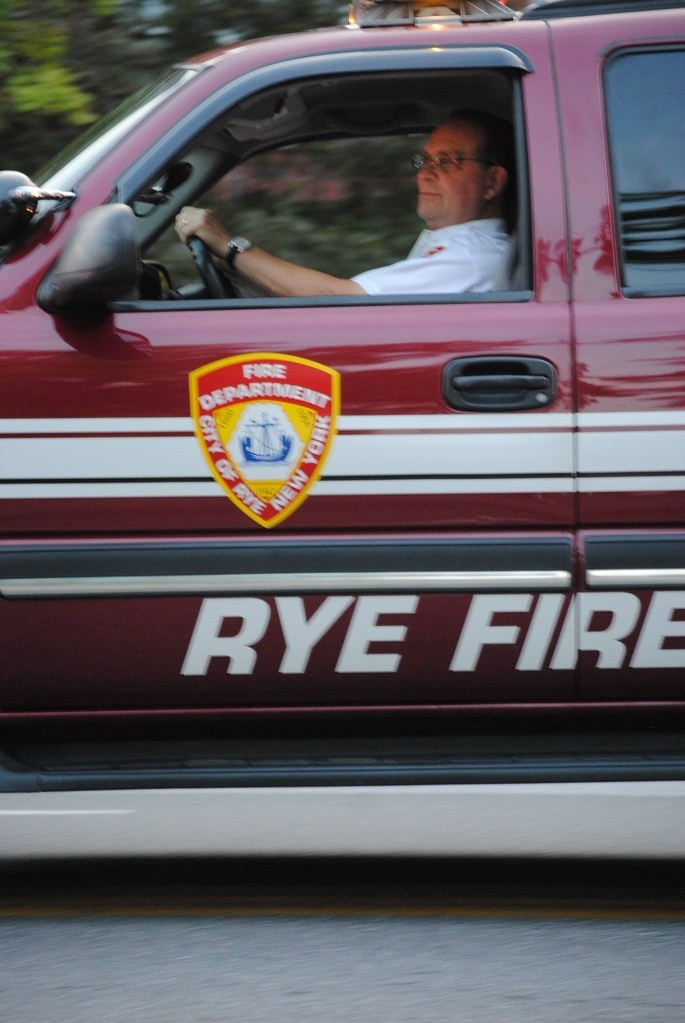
[175,112,516,296]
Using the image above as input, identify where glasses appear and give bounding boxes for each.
[412,151,489,174]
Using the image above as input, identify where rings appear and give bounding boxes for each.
[182,218,189,224]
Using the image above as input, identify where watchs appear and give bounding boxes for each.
[225,236,251,270]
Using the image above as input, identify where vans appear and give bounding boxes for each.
[1,1,685,868]
